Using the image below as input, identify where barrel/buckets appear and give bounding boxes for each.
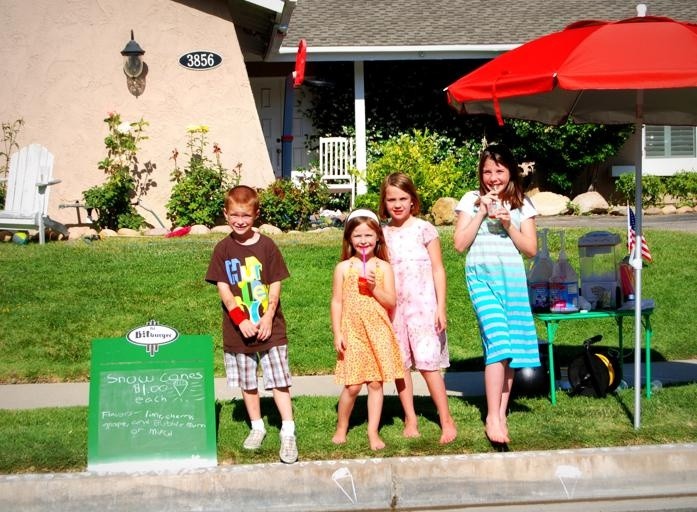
[526,255,553,313]
[548,252,580,313]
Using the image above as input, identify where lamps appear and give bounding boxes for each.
[119,30,147,78]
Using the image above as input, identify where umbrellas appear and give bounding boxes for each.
[444,2,697,429]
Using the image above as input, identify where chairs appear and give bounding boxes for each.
[319,135,355,209]
[0,142,71,246]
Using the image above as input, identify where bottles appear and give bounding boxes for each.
[615,380,628,393]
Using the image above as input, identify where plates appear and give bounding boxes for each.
[550,307,578,313]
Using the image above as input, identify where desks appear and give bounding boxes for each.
[536,297,655,407]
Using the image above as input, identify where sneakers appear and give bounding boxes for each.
[243,429,266,449]
[278,428,298,464]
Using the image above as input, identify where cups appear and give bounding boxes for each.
[643,380,663,395]
[357,275,370,296]
[488,202,500,219]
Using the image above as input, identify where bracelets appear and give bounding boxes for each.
[229,307,248,326]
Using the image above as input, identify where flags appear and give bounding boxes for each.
[627,204,654,264]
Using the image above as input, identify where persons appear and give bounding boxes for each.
[453,142,542,445]
[376,172,459,444]
[204,184,298,464]
[330,206,405,450]
